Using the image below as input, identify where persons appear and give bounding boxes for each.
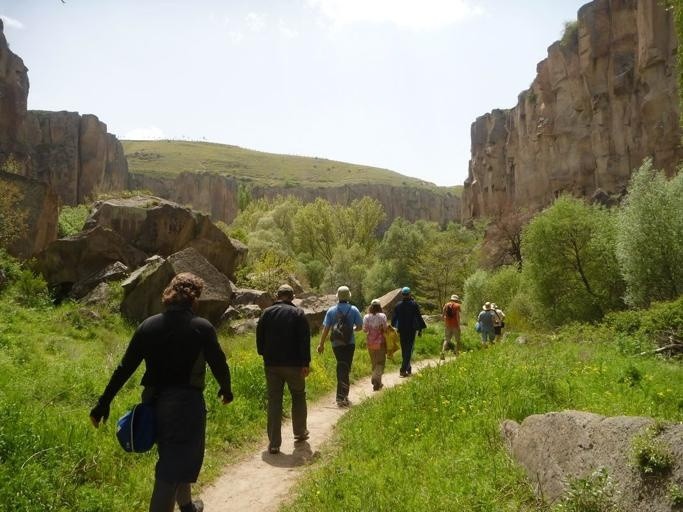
[439,295,461,360]
[90,272,233,512]
[363,299,388,391]
[256,283,310,454]
[317,286,363,406]
[491,303,505,337]
[392,286,426,377]
[478,302,496,344]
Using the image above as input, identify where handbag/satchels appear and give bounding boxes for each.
[475,322,481,332]
[117,404,156,453]
[383,325,399,354]
[502,321,505,327]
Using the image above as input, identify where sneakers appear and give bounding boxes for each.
[399,366,412,376]
[336,398,350,408]
[440,351,445,360]
[294,433,309,440]
[269,442,279,453]
[371,380,384,390]
[180,500,203,512]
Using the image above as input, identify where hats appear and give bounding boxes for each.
[402,287,410,294]
[371,299,380,305]
[336,286,351,301]
[451,295,459,301]
[482,301,498,311]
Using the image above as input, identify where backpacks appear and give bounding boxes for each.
[331,308,352,342]
[446,304,454,316]
